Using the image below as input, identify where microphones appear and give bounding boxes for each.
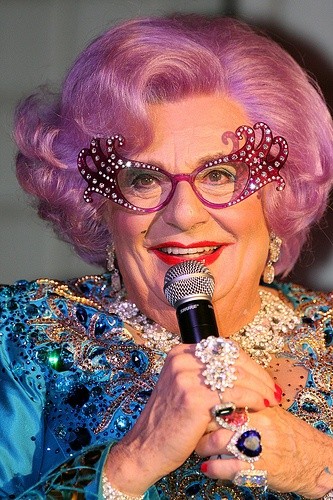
[163,261,219,343]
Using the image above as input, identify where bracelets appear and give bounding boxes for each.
[100,472,148,500]
[315,489,332,500]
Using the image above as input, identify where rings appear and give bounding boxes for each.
[194,333,268,490]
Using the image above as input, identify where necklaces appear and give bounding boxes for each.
[112,286,301,373]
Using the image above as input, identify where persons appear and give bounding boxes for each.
[0,12,333,500]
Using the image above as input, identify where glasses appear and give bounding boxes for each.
[76,121,290,213]
